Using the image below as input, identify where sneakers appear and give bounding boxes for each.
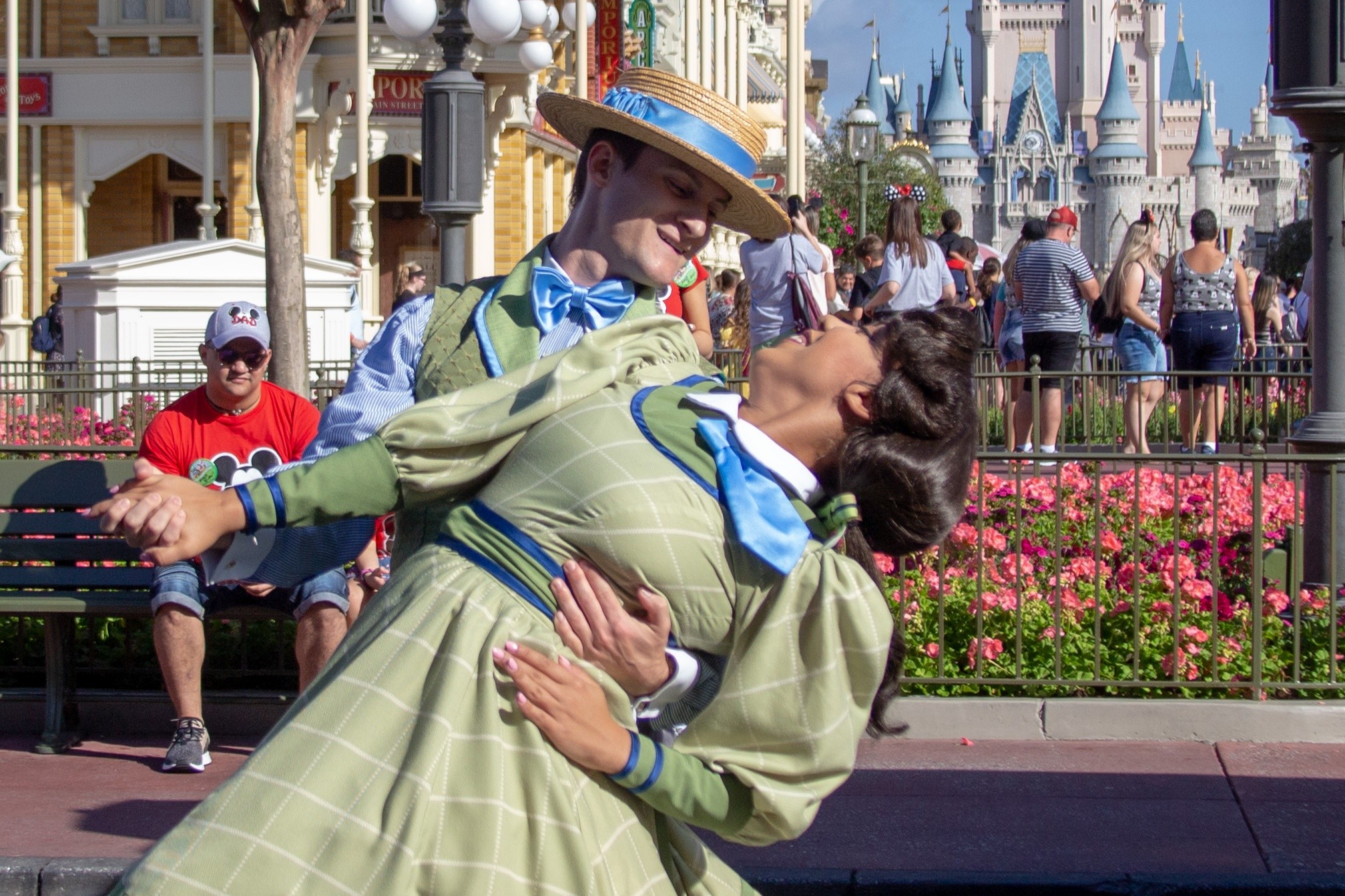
[1039,449,1077,468]
[1009,448,1035,466]
[1178,445,1196,454]
[1200,445,1216,455]
[161,717,212,772]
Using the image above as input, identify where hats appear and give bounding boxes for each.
[839,264,855,275]
[1047,207,1079,231]
[1021,219,1048,239]
[204,301,271,354]
[535,66,793,240]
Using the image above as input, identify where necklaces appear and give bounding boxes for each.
[203,391,261,417]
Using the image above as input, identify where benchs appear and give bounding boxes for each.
[0,445,292,755]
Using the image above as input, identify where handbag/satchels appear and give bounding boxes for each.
[1090,294,1124,333]
[785,235,829,335]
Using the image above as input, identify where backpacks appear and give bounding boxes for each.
[971,297,992,345]
[1281,305,1304,341]
[31,303,62,354]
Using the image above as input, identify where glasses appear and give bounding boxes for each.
[204,343,267,368]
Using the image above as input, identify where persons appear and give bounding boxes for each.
[337,250,368,357]
[1012,204,1100,469]
[1159,208,1260,468]
[1101,217,1170,459]
[973,255,1008,414]
[862,196,957,320]
[93,64,795,731]
[1235,249,1317,407]
[659,194,883,400]
[935,208,986,308]
[987,218,1048,462]
[135,301,350,775]
[1089,267,1120,397]
[42,284,67,409]
[78,299,984,896]
[391,261,428,315]
[344,510,398,627]
[947,237,982,311]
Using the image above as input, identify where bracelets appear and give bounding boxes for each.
[359,567,376,582]
[968,297,977,308]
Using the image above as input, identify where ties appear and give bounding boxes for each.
[696,417,812,578]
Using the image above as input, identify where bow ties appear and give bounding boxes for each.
[529,266,636,330]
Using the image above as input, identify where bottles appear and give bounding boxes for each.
[378,555,392,583]
